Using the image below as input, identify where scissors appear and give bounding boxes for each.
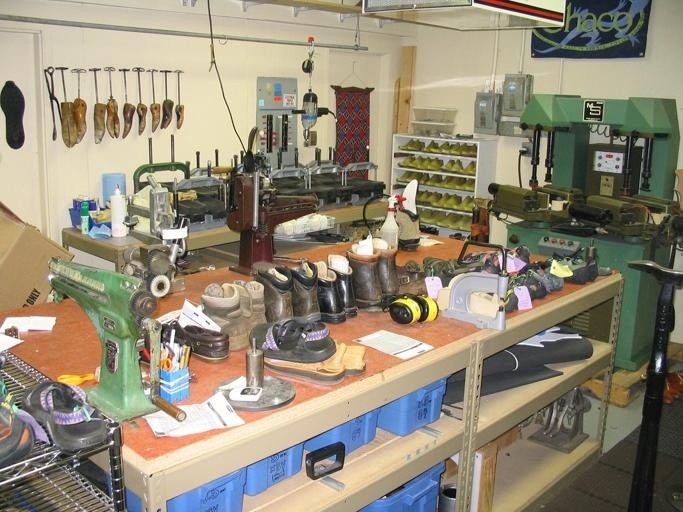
[160,358,172,371]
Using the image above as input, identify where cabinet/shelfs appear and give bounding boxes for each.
[390,134,497,239]
[0,350,125,512]
[1,237,626,512]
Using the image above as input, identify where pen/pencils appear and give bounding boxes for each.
[207,402,226,427]
[144,328,191,370]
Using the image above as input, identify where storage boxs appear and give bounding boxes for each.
[0,204,74,308]
[413,107,457,122]
[411,121,456,137]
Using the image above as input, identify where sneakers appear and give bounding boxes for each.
[396,244,597,311]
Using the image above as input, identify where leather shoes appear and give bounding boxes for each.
[183,320,229,364]
[326,266,357,319]
[316,267,346,324]
[416,207,473,232]
[398,139,477,157]
[415,190,474,213]
[395,172,474,192]
[397,155,476,176]
[159,319,194,352]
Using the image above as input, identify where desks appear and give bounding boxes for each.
[61,198,390,274]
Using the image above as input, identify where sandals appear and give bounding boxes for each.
[248,318,337,364]
[0,374,50,469]
[22,381,107,456]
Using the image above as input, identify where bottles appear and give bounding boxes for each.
[108,188,128,238]
[78,201,89,236]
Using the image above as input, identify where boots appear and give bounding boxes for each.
[255,267,295,323]
[291,260,322,324]
[197,282,250,352]
[346,247,384,313]
[376,245,400,297]
[234,280,267,332]
